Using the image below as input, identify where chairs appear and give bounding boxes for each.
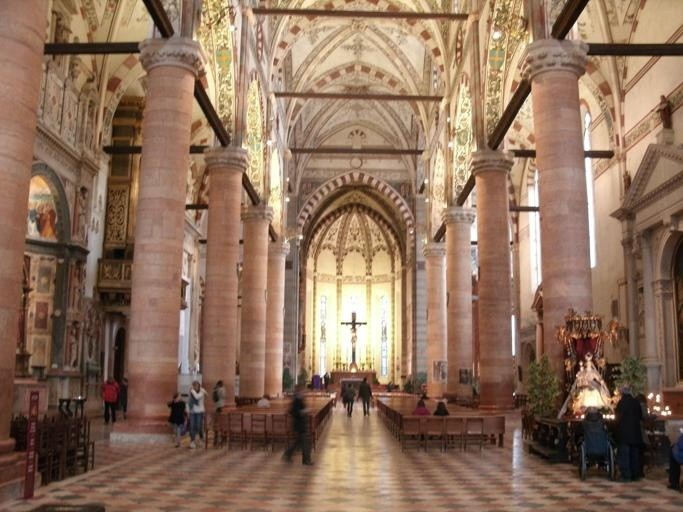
[203,412,317,455]
[403,416,483,453]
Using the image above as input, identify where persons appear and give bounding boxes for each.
[341,377,372,416]
[101,374,128,425]
[667,433,683,493]
[410,400,450,416]
[256,393,271,407]
[386,381,392,392]
[280,382,315,467]
[323,372,331,390]
[212,379,227,411]
[567,383,646,483]
[168,378,207,448]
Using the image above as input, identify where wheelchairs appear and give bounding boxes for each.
[567,429,619,482]
